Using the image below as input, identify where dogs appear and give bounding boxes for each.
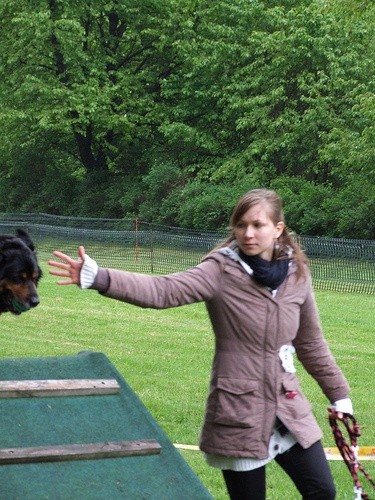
[0,228,42,315]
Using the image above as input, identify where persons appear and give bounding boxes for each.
[47,189,354,500]
